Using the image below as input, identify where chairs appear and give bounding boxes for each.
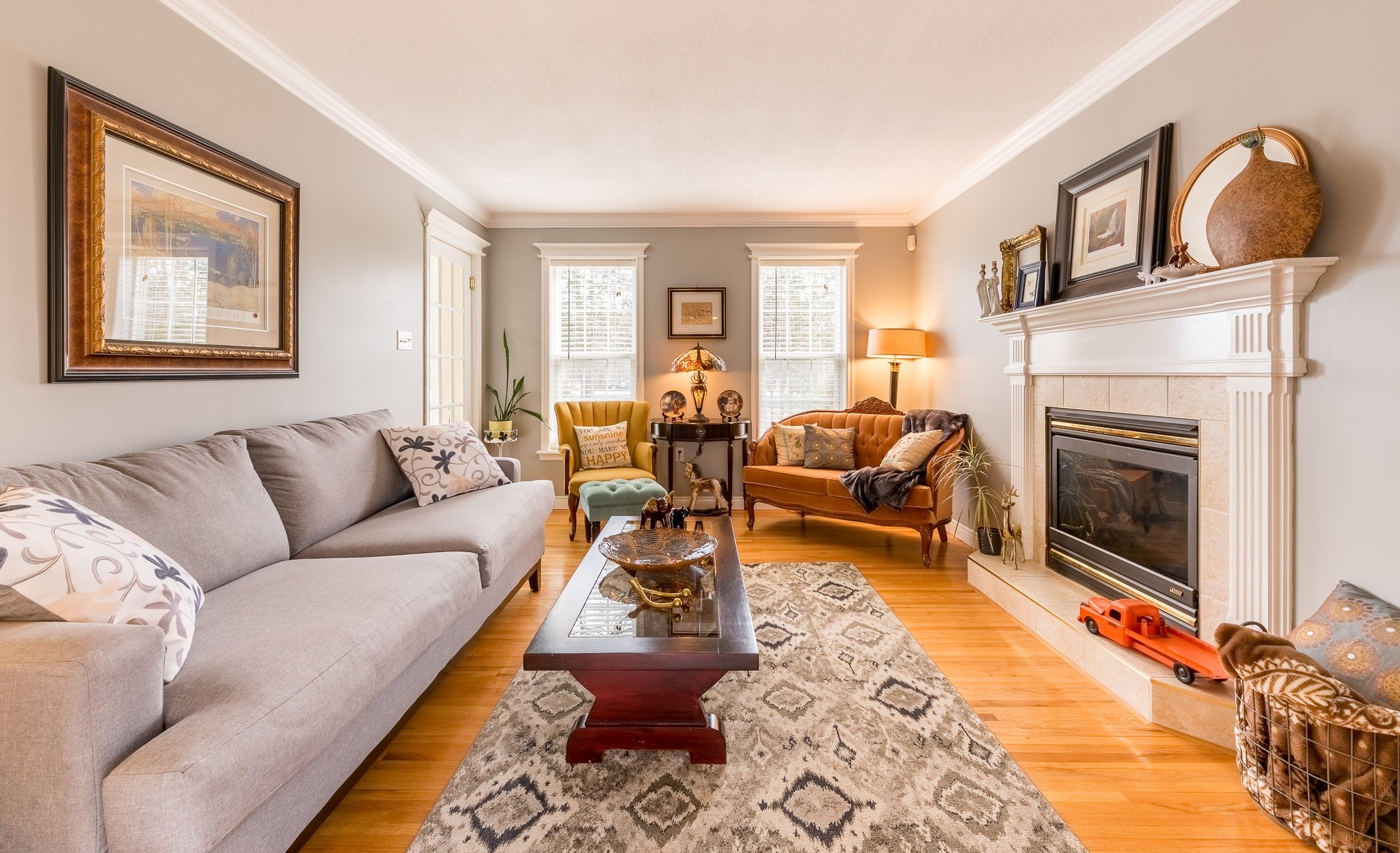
[1058,450,1162,523]
[554,401,658,542]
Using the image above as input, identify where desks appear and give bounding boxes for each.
[650,418,751,518]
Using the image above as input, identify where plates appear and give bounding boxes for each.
[598,526,720,572]
[716,390,744,417]
[660,390,687,418]
[598,566,715,604]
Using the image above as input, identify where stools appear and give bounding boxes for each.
[579,478,668,544]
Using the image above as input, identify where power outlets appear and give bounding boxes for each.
[675,447,684,462]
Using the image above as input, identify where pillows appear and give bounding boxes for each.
[1,481,205,689]
[772,421,943,472]
[1285,581,1399,714]
[379,421,512,506]
[572,421,633,471]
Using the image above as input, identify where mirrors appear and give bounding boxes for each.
[1170,127,1310,275]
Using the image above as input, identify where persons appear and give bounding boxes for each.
[977,261,1003,318]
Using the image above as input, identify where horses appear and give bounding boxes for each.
[683,463,730,513]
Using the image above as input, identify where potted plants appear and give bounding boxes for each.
[1058,453,1135,528]
[487,329,552,439]
[930,425,1005,556]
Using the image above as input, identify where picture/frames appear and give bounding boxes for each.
[47,66,301,383]
[668,287,726,339]
[1000,123,1173,313]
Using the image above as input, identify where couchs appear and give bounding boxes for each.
[1,408,556,853]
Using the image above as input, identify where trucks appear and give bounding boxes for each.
[1076,595,1237,685]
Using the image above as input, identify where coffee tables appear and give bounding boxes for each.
[523,516,759,764]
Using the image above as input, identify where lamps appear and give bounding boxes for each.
[669,341,727,423]
[866,329,927,407]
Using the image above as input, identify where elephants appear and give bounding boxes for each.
[668,506,688,529]
[639,491,675,529]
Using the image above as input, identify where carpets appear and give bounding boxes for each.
[405,562,1090,853]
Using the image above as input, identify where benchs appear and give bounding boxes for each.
[744,397,968,568]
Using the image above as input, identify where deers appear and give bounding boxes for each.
[998,484,1025,570]
